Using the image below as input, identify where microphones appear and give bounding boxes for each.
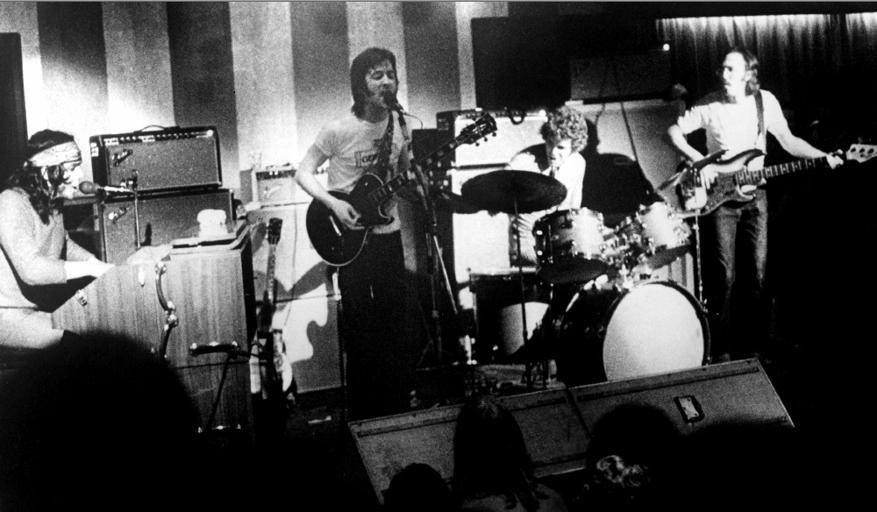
[384,93,401,114]
[80,181,132,194]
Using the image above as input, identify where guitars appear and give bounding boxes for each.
[305,110,497,267]
[249,218,293,400]
[675,136,877,217]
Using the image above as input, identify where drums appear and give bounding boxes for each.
[614,201,691,276]
[532,207,614,282]
[556,273,712,383]
[470,265,554,365]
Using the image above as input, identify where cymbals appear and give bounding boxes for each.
[461,171,567,215]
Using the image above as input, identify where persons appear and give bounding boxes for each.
[293,42,451,419]
[663,47,845,363]
[0,127,113,350]
[501,104,592,394]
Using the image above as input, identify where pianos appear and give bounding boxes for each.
[50,219,258,428]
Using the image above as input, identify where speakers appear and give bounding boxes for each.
[568,358,797,451]
[346,389,599,512]
